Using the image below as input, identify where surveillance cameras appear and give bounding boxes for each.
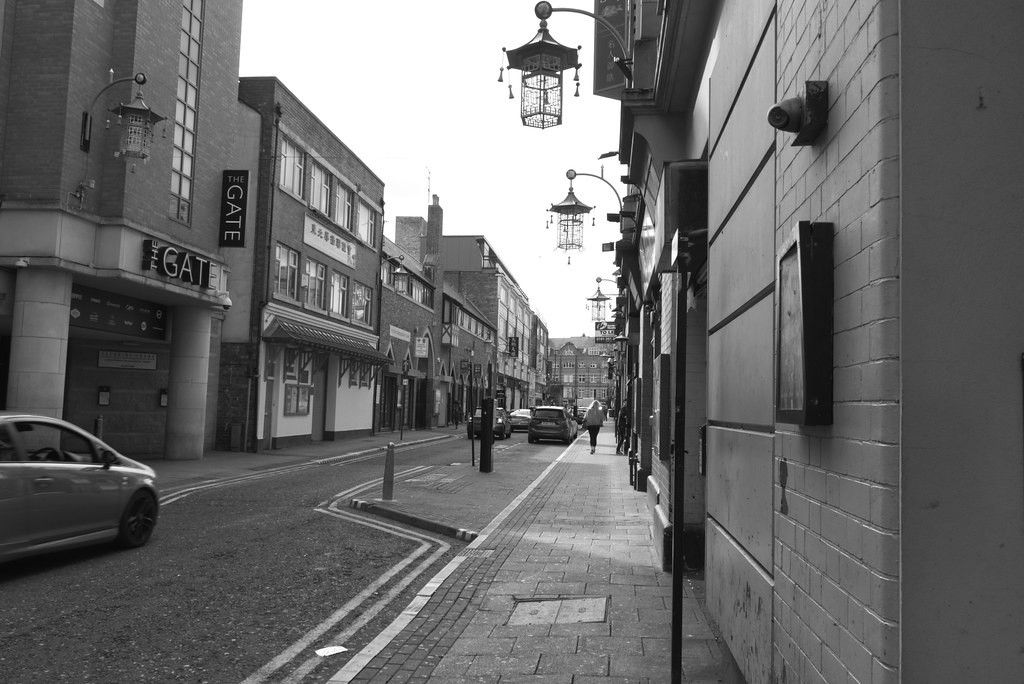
[766,96,801,133]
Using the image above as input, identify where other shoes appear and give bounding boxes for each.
[590,446,595,454]
[616,452,623,456]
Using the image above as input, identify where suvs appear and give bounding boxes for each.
[529,406,580,446]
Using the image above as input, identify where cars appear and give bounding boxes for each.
[508,408,534,433]
[563,406,590,429]
[468,406,512,441]
[1,411,161,565]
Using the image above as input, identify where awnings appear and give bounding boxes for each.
[262,317,394,389]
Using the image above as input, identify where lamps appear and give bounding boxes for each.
[472,331,493,355]
[546,168,623,265]
[80,73,168,174]
[585,277,620,322]
[381,255,410,297]
[498,1,634,129]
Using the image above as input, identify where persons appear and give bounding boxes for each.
[603,405,608,421]
[453,400,462,429]
[583,400,604,454]
[616,406,629,456]
